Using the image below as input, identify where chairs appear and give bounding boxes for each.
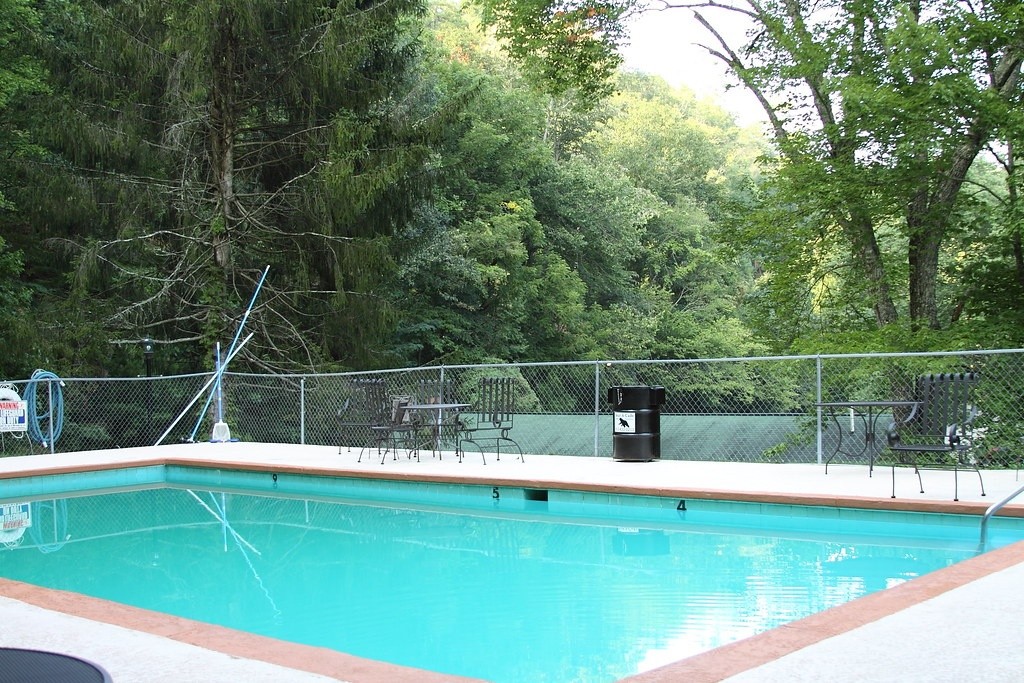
[337,377,524,464]
[890,373,986,501]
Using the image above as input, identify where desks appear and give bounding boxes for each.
[813,402,922,478]
[400,403,473,462]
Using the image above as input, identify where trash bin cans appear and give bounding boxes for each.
[606,385,667,463]
